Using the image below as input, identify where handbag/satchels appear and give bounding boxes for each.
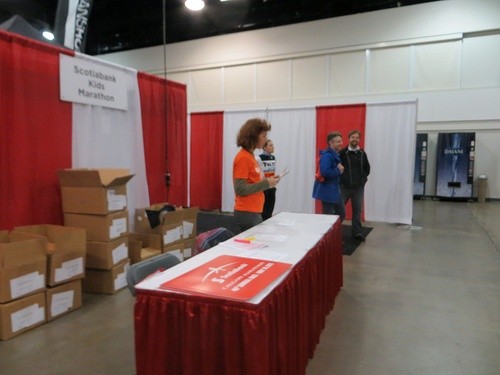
[316,168,325,181]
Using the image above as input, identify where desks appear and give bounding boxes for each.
[132,212,343,375]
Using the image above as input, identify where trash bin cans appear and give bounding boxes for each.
[477,174,488,203]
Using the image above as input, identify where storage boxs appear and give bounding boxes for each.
[0,167,200,341]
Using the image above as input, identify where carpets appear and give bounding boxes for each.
[198,213,373,256]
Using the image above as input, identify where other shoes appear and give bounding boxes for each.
[354,235,365,241]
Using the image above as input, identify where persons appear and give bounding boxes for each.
[259,140,277,221]
[234,118,279,231]
[313,133,345,224]
[339,130,371,241]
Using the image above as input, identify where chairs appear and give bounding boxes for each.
[192,227,233,256]
[126,253,181,296]
